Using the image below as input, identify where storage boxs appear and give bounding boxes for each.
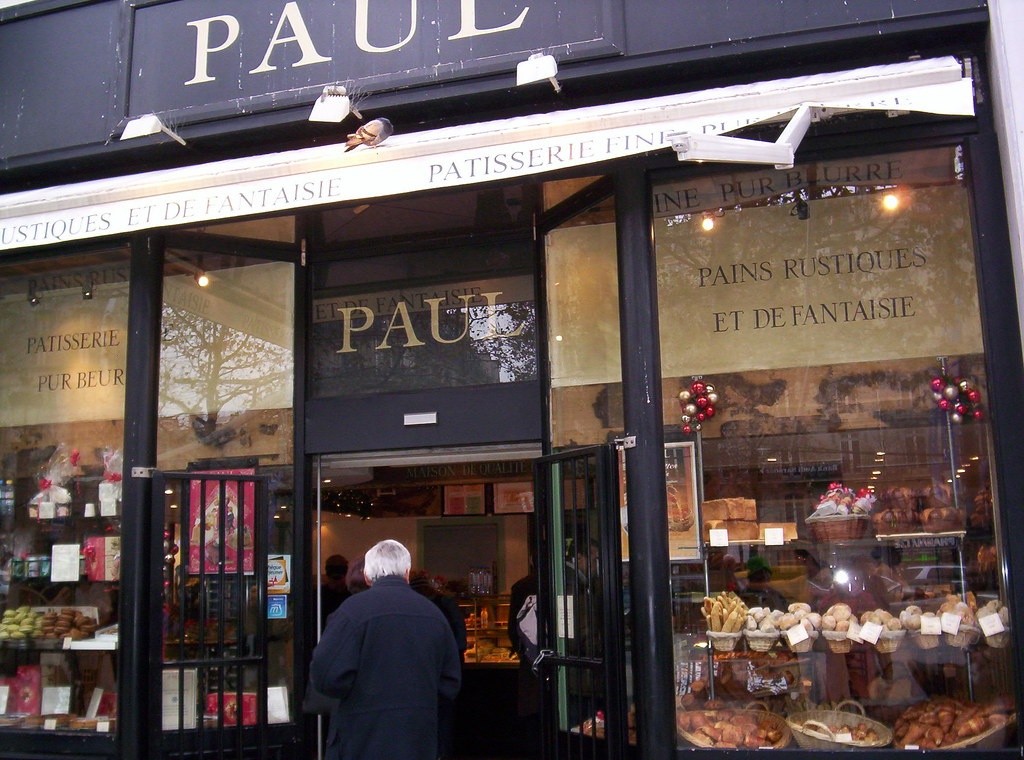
[84,535,121,582]
[205,692,255,727]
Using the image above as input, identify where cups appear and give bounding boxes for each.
[11,555,87,577]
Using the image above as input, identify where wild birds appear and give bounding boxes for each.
[344,117,393,153]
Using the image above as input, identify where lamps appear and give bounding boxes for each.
[790,197,810,221]
[701,212,715,230]
[194,259,209,287]
[516,53,561,94]
[309,86,362,123]
[120,115,187,146]
[881,191,900,211]
[26,281,40,308]
[80,274,93,299]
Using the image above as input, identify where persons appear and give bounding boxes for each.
[303,539,461,760]
[320,553,370,637]
[507,538,607,760]
[678,544,929,720]
[217,585,294,691]
[5,536,116,709]
[412,571,466,760]
[174,565,208,621]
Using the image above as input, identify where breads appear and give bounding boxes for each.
[891,697,1016,750]
[742,592,1010,632]
[582,710,636,744]
[871,483,965,534]
[0,606,99,641]
[700,498,799,544]
[701,590,750,632]
[797,719,880,743]
[676,647,805,751]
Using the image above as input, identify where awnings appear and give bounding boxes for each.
[0,55,975,254]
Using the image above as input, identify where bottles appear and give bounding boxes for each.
[467,568,493,597]
[0,479,16,516]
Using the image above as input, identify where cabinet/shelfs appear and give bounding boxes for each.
[0,513,291,760]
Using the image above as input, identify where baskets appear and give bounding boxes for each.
[827,639,851,653]
[786,700,894,748]
[946,629,979,647]
[873,513,916,535]
[676,700,792,750]
[984,628,1011,648]
[894,713,1019,751]
[785,636,815,652]
[712,637,737,651]
[874,638,902,654]
[914,632,939,649]
[920,510,960,533]
[747,636,775,652]
[805,514,872,543]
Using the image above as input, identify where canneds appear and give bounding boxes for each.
[10,555,52,578]
